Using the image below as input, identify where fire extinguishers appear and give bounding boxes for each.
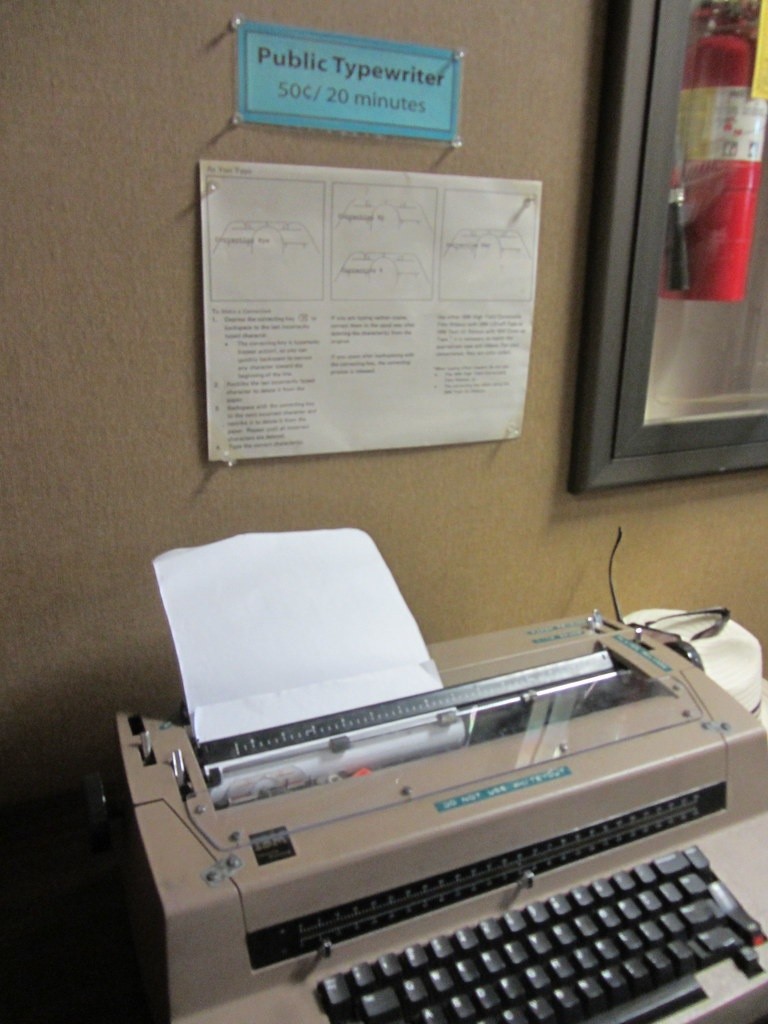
[658,1,767,301]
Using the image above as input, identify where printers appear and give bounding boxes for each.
[114,612,768,1024]
